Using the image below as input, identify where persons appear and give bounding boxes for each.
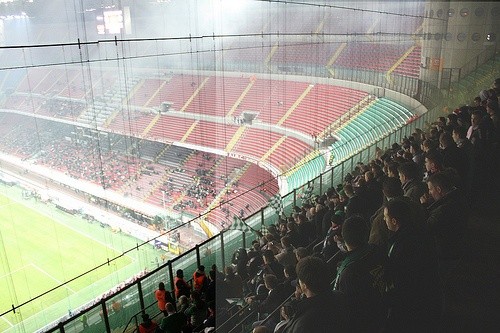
[382,196,431,332]
[78,188,344,333]
[275,253,352,332]
[332,215,389,332]
[418,171,469,278]
[0,87,266,251]
[332,74,500,235]
